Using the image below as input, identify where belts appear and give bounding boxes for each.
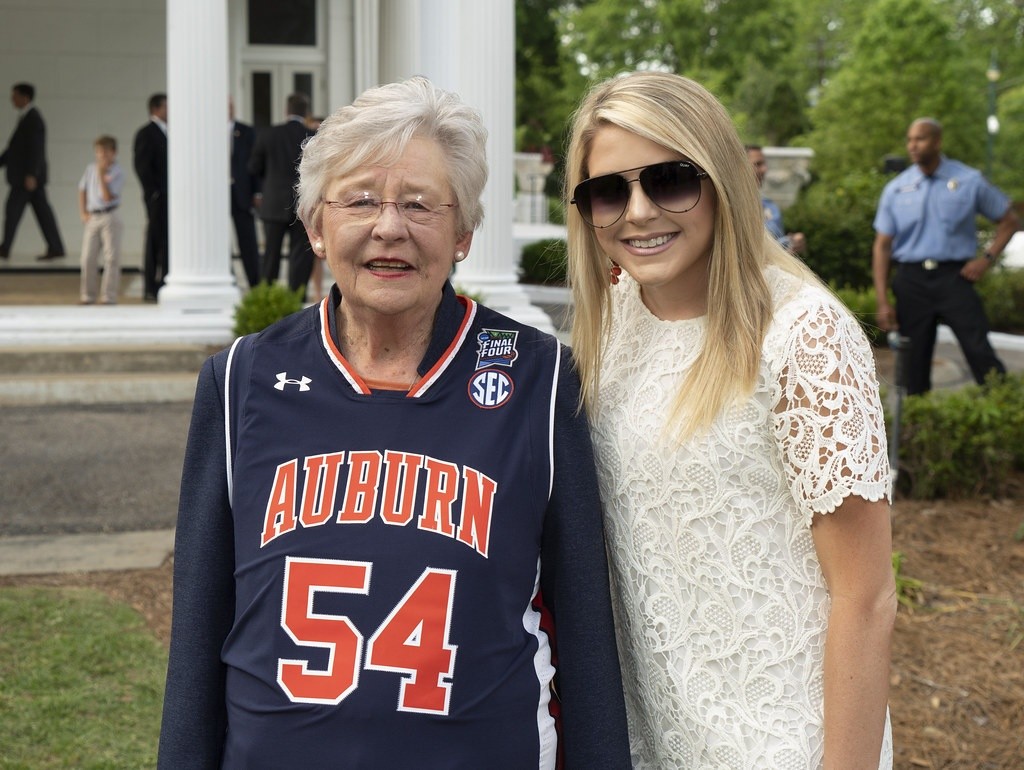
[904,259,961,271]
[92,206,117,213]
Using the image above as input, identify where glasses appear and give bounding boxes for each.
[320,190,459,219]
[752,161,767,167]
[570,161,710,228]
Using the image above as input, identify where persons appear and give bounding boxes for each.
[0,80,66,261]
[558,69,895,770]
[247,93,317,303]
[300,117,329,298]
[133,93,169,301]
[872,117,1020,397]
[78,135,125,303]
[741,142,805,255]
[225,94,261,288]
[153,74,633,770]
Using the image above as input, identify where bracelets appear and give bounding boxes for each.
[984,252,996,263]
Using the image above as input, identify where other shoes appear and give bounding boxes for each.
[1,246,7,257]
[36,248,63,260]
[144,289,159,301]
